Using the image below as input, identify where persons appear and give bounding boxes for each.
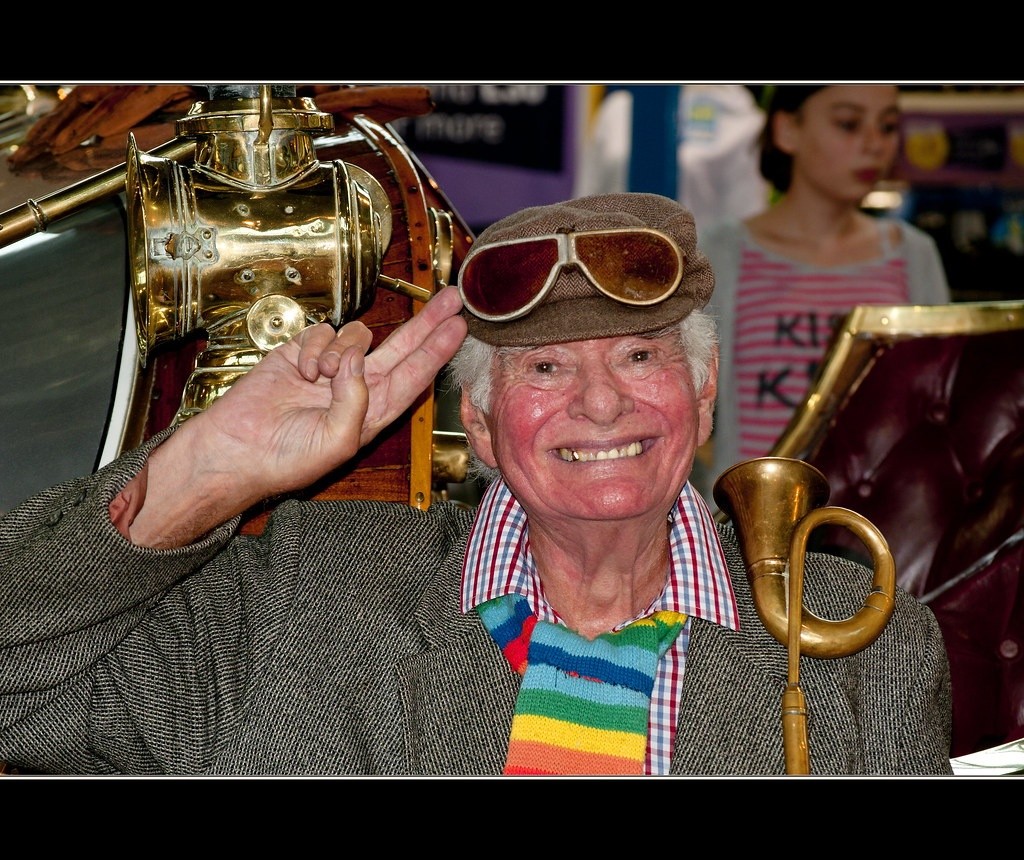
[700,80,951,517]
[1,189,954,776]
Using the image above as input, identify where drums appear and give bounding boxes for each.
[84,108,472,538]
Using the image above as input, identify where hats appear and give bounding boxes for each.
[456,193,713,347]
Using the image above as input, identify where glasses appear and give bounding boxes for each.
[457,229,687,322]
